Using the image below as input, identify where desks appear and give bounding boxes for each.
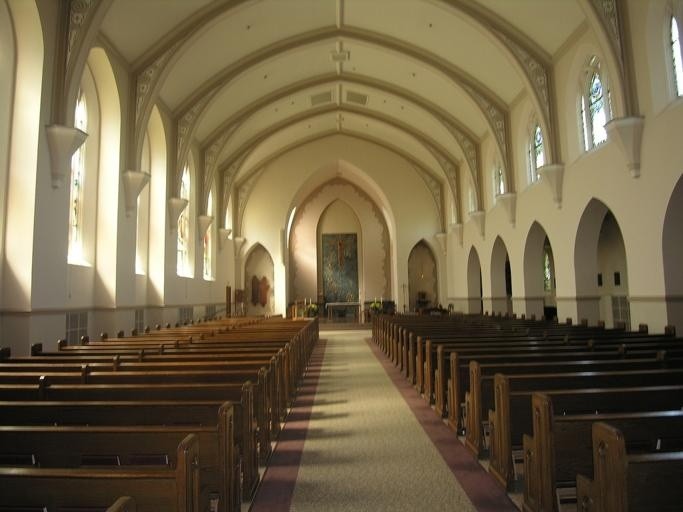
[325,302,361,324]
